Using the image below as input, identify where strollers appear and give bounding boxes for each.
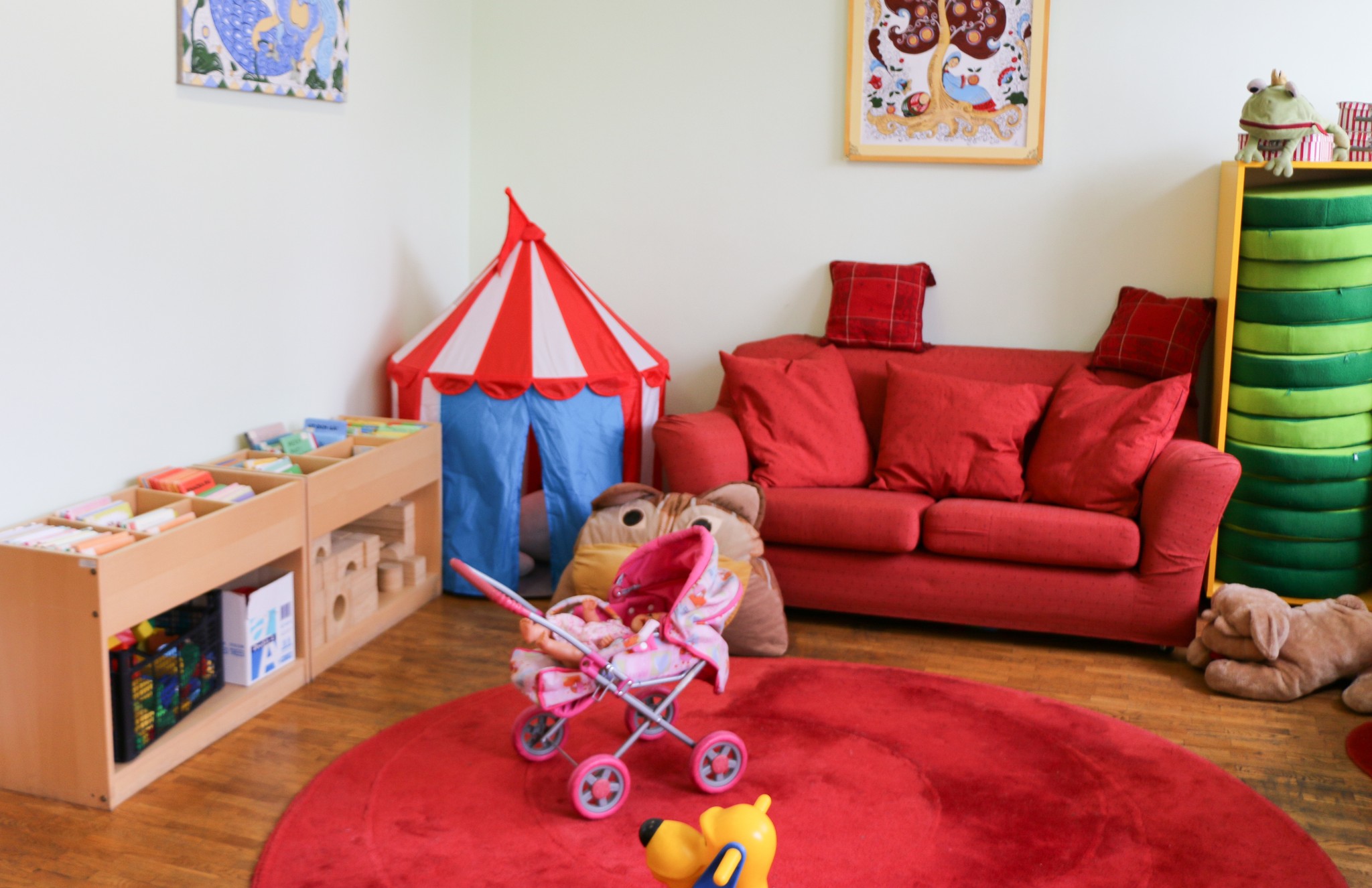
[449,524,749,820]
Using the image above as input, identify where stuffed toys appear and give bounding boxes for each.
[1186,583,1372,713]
[1234,67,1351,178]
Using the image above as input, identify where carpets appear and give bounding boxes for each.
[247,653,1348,888]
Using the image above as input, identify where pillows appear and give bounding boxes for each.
[1015,364,1194,519]
[869,358,1056,505]
[816,260,936,355]
[1086,285,1218,381]
[716,342,876,490]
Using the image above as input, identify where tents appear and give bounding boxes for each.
[385,187,671,598]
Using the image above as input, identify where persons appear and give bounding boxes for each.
[521,599,671,670]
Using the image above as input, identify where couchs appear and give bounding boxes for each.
[649,332,1247,660]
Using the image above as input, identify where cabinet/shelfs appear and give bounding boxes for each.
[0,415,444,813]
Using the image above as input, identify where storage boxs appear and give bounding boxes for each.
[1204,161,1372,613]
[217,571,297,689]
[110,602,227,764]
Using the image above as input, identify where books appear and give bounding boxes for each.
[0,415,429,556]
